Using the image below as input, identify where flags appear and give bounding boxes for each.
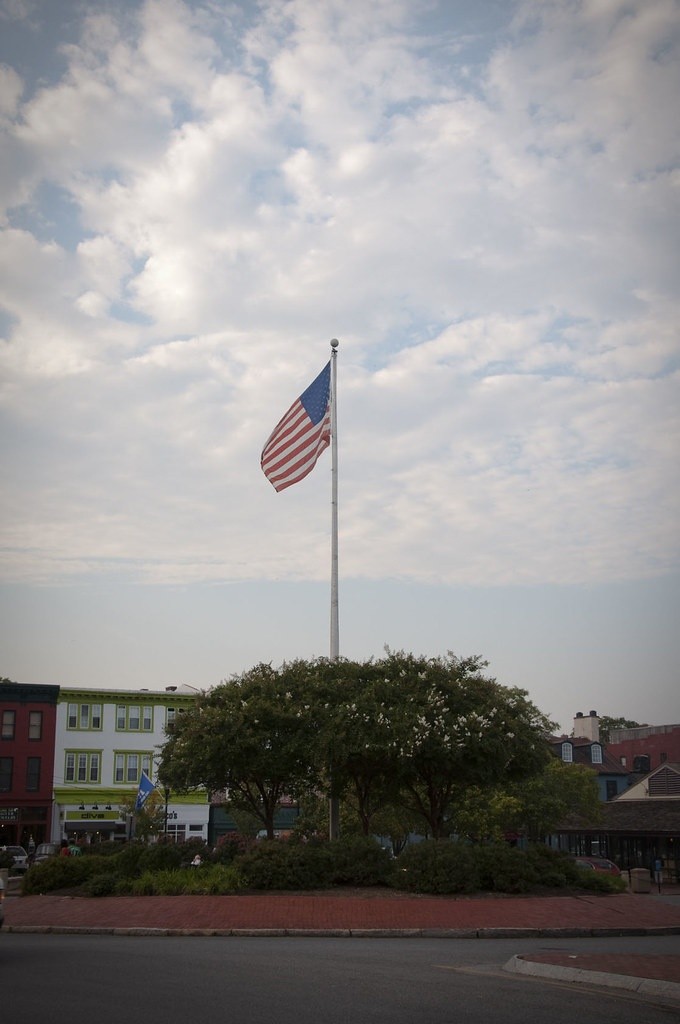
[135,770,155,813]
[260,354,334,493]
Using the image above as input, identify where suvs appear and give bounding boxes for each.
[0,845,29,873]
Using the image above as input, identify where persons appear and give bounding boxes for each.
[649,856,665,880]
[56,839,72,860]
[68,838,82,857]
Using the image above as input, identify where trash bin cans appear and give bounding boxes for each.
[630,868,651,894]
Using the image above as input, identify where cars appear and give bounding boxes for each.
[32,843,57,866]
